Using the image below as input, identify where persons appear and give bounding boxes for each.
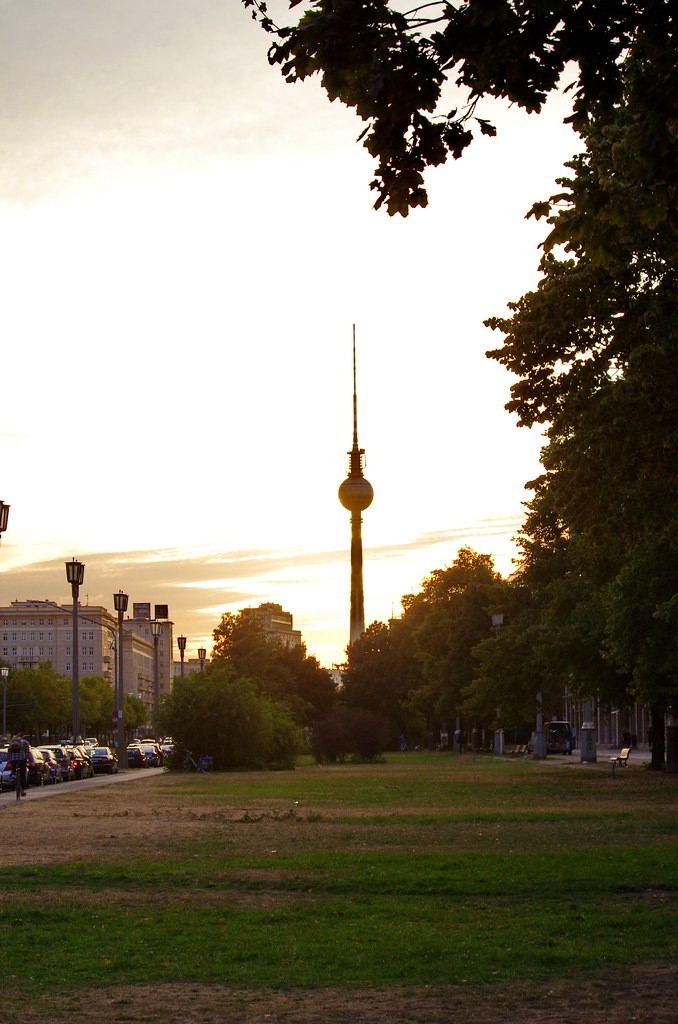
[399,734,406,752]
[8,733,34,796]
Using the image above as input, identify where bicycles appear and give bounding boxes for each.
[178,748,214,774]
[11,763,22,801]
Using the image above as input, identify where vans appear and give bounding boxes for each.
[530,721,575,755]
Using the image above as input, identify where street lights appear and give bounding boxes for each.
[148,619,164,714]
[115,586,127,770]
[196,647,206,672]
[1,665,10,735]
[62,557,87,738]
[175,634,189,677]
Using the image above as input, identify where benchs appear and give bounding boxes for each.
[512,744,527,757]
[608,748,632,768]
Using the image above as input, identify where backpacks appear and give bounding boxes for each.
[9,737,22,754]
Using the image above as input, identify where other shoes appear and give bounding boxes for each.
[8,777,16,784]
[21,792,26,796]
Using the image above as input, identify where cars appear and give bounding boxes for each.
[92,747,118,774]
[124,731,176,755]
[144,746,161,767]
[55,735,100,753]
[0,744,95,790]
[126,747,141,767]
